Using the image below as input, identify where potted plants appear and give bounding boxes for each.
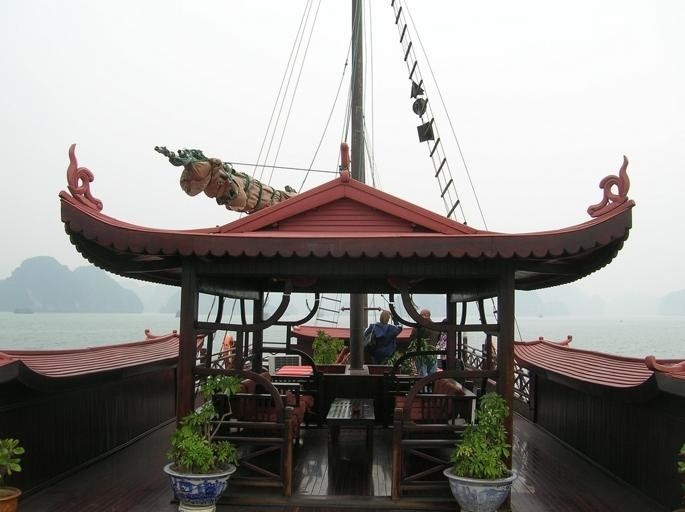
[161,371,244,512]
[0,437,25,512]
[441,388,518,512]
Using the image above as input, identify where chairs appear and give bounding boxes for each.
[176,347,316,497]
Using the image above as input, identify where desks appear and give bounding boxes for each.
[326,396,376,465]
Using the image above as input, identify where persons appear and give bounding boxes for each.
[363,310,402,363]
[410,309,438,394]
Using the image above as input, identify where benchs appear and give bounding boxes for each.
[391,350,511,503]
